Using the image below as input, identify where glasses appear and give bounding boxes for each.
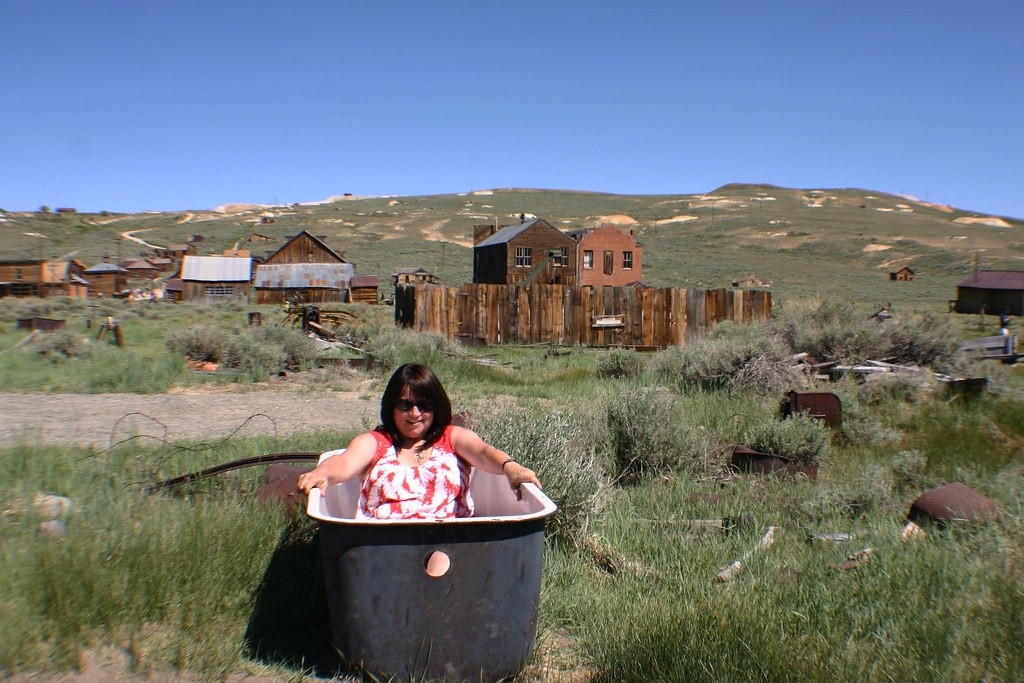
[392,398,435,411]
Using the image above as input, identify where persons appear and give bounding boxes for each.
[1000,306,1013,335]
[870,300,892,320]
[297,362,543,521]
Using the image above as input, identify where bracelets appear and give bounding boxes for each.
[502,459,517,473]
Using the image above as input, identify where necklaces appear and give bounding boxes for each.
[414,452,425,465]
